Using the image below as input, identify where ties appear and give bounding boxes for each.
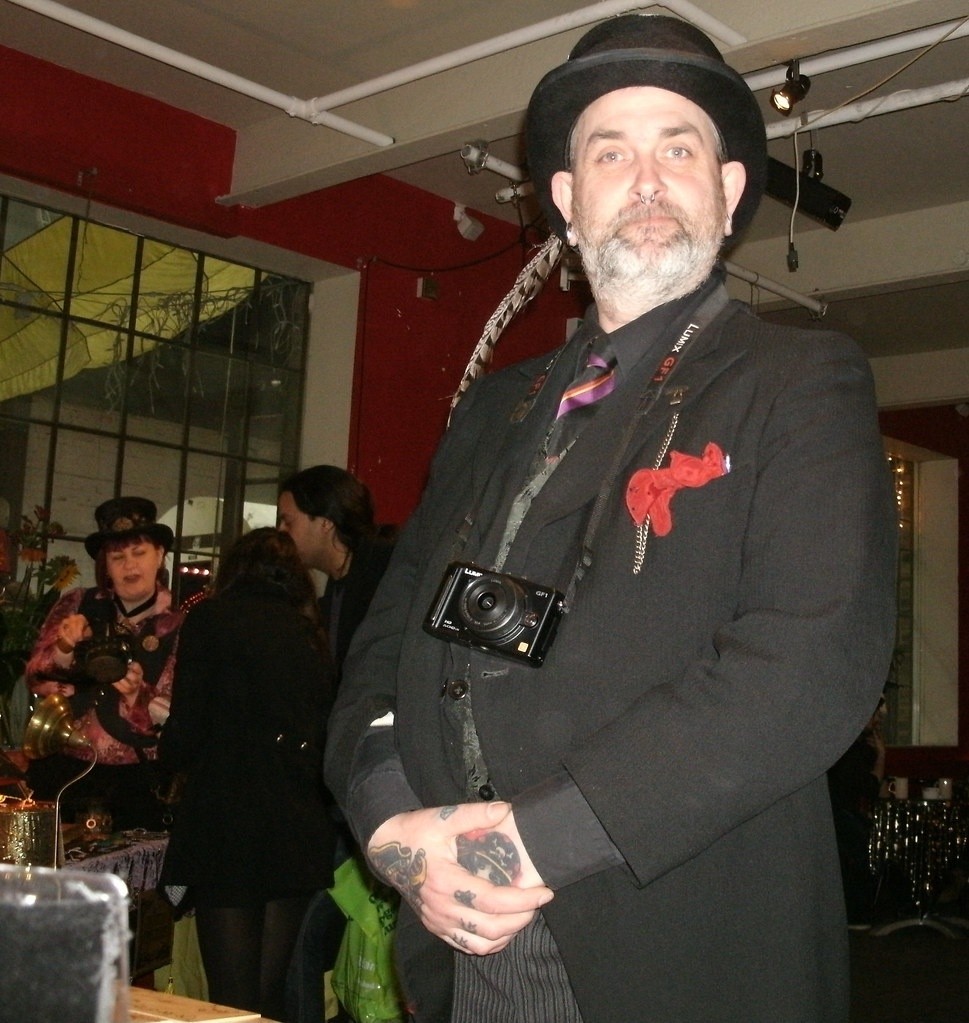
[557,343,614,422]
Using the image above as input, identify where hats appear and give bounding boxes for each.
[86,496,176,557]
[521,15,767,251]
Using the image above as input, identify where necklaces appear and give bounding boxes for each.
[110,584,159,637]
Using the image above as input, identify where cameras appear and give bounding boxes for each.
[423,561,565,669]
[74,633,136,684]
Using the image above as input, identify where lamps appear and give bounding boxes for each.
[769,58,812,116]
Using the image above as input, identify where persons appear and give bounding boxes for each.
[26,498,184,834]
[273,465,403,684]
[153,528,337,1022]
[320,13,896,1021]
